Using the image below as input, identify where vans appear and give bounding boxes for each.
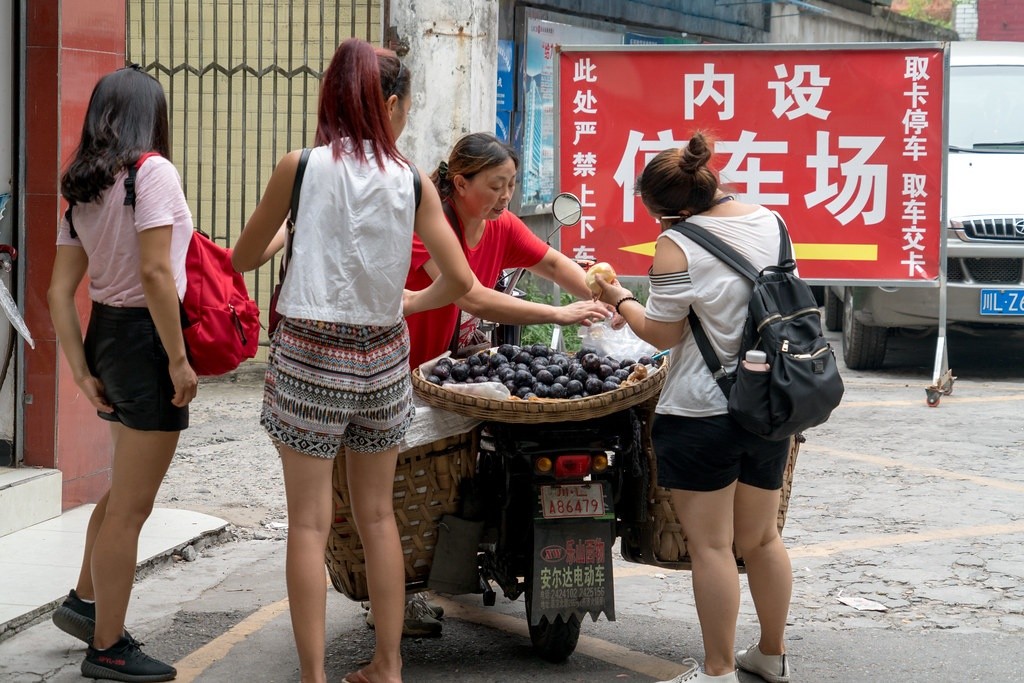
[816,40,1024,369]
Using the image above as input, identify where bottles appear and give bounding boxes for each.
[741,351,770,372]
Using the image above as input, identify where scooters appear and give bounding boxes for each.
[455,193,621,666]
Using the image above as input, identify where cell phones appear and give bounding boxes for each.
[660,215,688,233]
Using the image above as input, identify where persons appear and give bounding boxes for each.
[229,36,474,683]
[47,68,199,682]
[358,130,614,635]
[596,132,793,683]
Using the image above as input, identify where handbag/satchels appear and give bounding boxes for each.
[266,143,312,340]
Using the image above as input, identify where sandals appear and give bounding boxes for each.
[342,669,376,683]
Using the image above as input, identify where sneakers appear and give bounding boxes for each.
[736,642,790,683]
[360,586,445,620]
[655,665,740,683]
[81,633,177,682]
[53,589,142,650]
[366,598,442,635]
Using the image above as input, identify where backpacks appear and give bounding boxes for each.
[68,151,260,379]
[666,209,845,441]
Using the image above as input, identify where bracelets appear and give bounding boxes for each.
[615,296,640,314]
